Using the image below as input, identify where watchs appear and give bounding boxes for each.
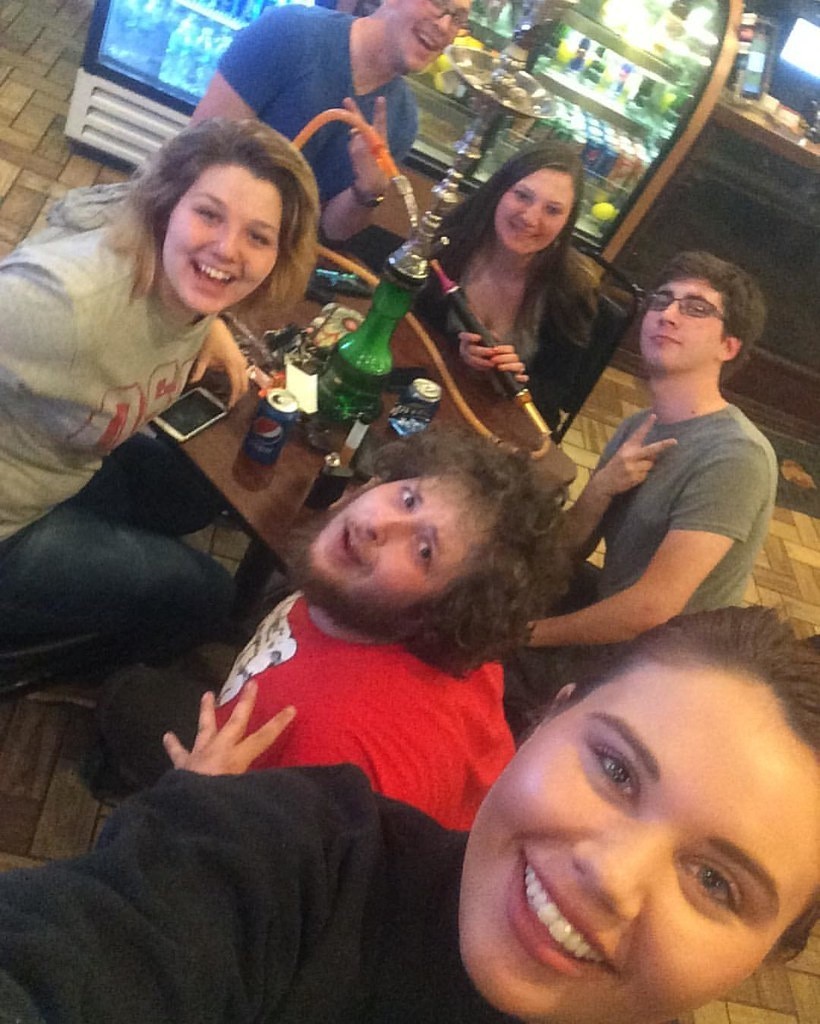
[350,182,384,208]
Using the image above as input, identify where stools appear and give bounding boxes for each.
[0,609,128,704]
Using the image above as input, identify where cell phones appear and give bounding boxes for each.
[152,387,229,443]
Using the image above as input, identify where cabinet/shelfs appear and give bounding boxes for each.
[61,0,744,298]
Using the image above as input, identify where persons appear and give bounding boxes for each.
[2,2,820,1023]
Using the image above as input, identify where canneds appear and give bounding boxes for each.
[501,92,653,194]
[243,388,299,466]
[389,379,441,438]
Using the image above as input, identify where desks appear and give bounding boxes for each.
[170,254,577,632]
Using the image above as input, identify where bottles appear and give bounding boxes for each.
[106,0,287,104]
[565,38,634,95]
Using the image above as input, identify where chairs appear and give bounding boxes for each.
[532,236,638,443]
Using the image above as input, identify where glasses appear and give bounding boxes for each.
[643,292,728,324]
[423,0,471,28]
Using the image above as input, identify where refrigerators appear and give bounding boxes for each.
[61,1,339,170]
